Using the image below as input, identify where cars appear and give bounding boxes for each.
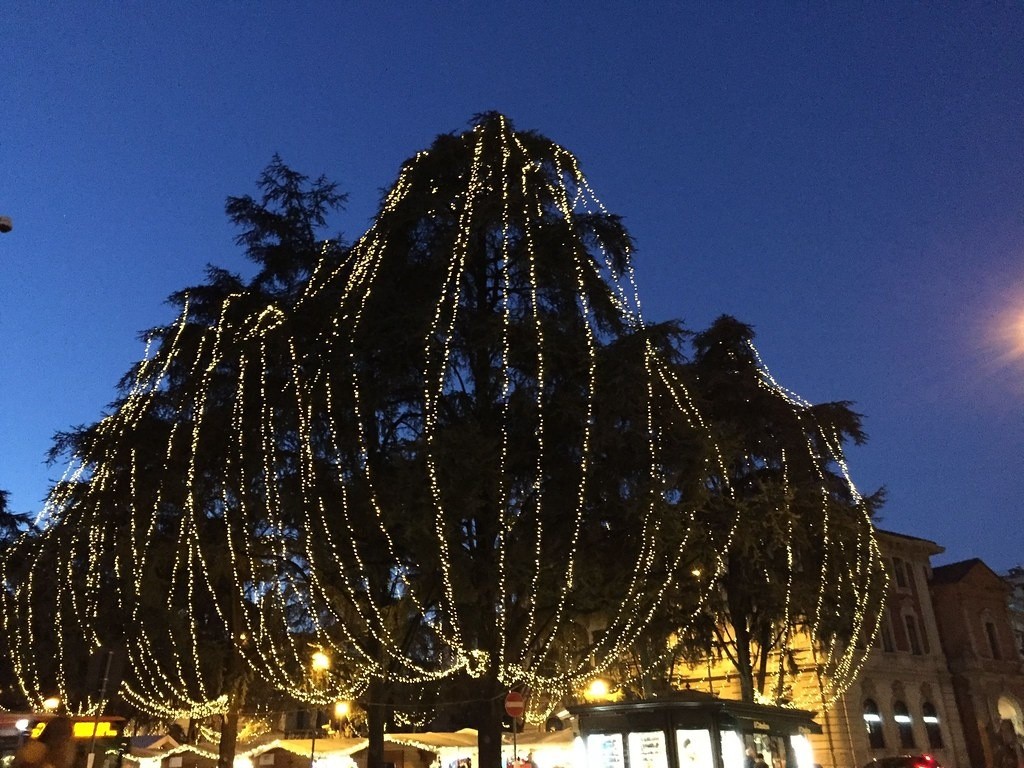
[862,754,944,768]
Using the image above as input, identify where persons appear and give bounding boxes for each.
[526,754,537,768]
[743,748,756,768]
[754,754,769,768]
[11,716,73,768]
